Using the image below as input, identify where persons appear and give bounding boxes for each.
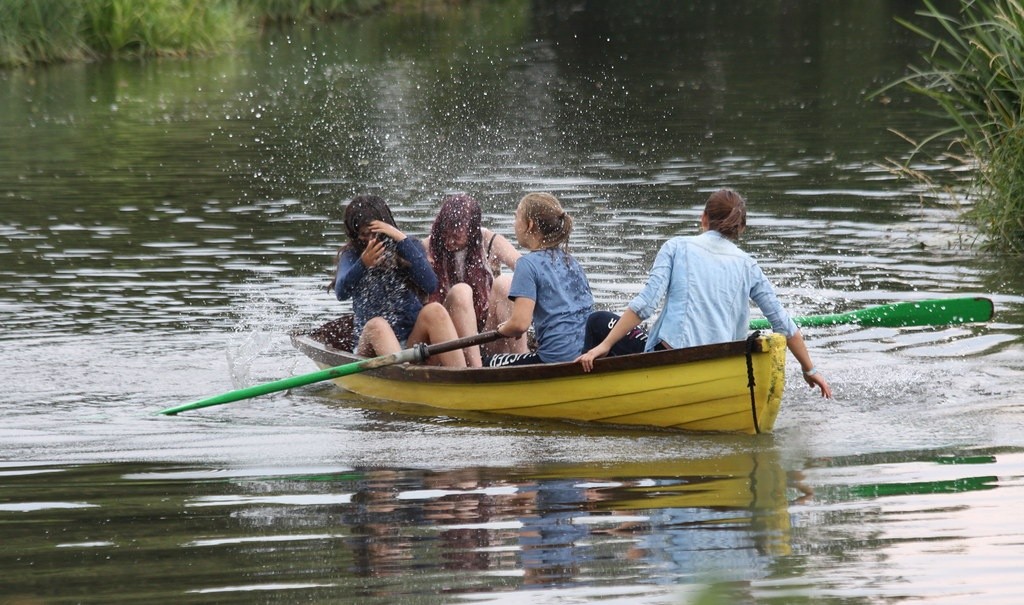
[420,196,530,367]
[483,192,596,367]
[573,190,833,399]
[328,196,467,368]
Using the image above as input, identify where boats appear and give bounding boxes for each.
[289,314,790,437]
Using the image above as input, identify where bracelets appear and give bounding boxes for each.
[802,367,816,375]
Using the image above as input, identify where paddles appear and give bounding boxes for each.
[159,327,505,416]
[750,296,995,330]
[814,475,1002,498]
